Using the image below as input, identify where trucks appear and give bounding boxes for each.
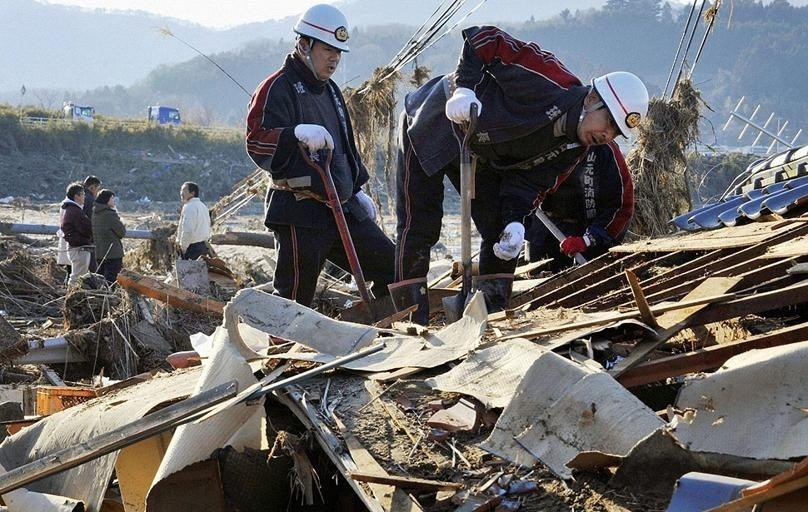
[106,104,183,128]
[23,101,96,128]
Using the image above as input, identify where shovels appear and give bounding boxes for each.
[296,140,397,327]
[440,102,489,323]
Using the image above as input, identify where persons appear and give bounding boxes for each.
[54,230,74,292]
[174,180,212,262]
[385,19,651,335]
[245,3,396,377]
[517,135,634,296]
[90,187,127,287]
[83,175,102,273]
[60,182,92,295]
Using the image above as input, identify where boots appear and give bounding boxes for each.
[470,272,514,314]
[387,277,431,328]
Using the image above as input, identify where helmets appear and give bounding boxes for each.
[591,71,650,139]
[292,2,351,52]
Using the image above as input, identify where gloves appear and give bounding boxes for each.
[444,87,483,126]
[355,187,377,222]
[491,219,526,261]
[558,233,592,257]
[293,121,336,155]
